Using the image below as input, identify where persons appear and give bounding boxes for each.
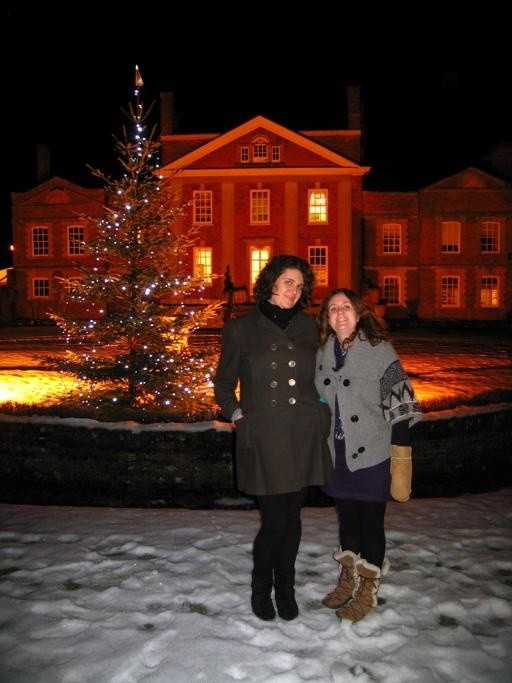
[215,254,334,622]
[314,287,417,622]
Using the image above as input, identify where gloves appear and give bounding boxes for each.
[389,443,416,504]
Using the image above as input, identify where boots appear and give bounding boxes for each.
[250,518,305,622]
[321,545,391,624]
